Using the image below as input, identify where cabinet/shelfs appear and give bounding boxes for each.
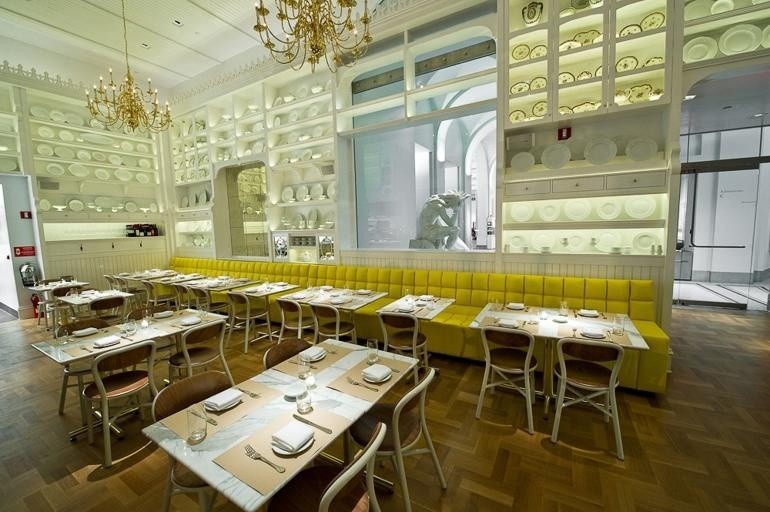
[501,0,670,255]
[25,93,160,223]
[674,0,770,72]
[0,84,24,175]
[165,57,338,261]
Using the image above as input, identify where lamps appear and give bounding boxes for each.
[253,0,374,74]
[85,0,171,133]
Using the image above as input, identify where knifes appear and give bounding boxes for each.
[288,359,318,369]
[189,407,218,425]
[291,411,335,439]
[382,363,400,374]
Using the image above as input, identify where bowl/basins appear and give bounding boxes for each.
[281,378,307,396]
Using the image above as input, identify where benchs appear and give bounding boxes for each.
[167,255,671,395]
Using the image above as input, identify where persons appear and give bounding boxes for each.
[417,190,463,251]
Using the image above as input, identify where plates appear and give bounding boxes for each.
[270,430,313,458]
[1,84,340,253]
[34,268,372,359]
[393,293,440,321]
[292,343,330,363]
[197,394,242,414]
[503,2,769,261]
[485,300,629,343]
[362,372,393,383]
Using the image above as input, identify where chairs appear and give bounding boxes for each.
[474,326,539,435]
[549,337,626,462]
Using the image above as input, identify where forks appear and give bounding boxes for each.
[244,441,285,478]
[344,372,381,394]
[239,385,263,405]
[316,345,338,353]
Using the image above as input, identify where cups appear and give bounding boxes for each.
[295,389,312,414]
[182,403,210,443]
[364,337,379,366]
[295,353,309,380]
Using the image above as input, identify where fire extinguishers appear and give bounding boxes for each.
[31,294,44,318]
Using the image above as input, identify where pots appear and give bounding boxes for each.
[20,261,40,287]
